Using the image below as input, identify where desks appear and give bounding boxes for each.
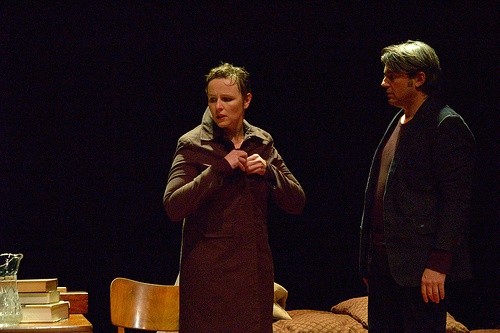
[0,313,93,333]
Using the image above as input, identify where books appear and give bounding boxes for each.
[0,278,70,323]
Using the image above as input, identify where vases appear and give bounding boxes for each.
[1,253,23,327]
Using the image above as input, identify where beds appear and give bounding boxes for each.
[272,310,368,333]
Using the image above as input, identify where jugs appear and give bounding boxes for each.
[0,253,24,329]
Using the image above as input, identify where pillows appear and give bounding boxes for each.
[331,297,470,333]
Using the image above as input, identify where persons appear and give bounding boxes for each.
[162,62,306,333]
[358,39,478,333]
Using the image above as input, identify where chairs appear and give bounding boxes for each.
[110,278,179,333]
